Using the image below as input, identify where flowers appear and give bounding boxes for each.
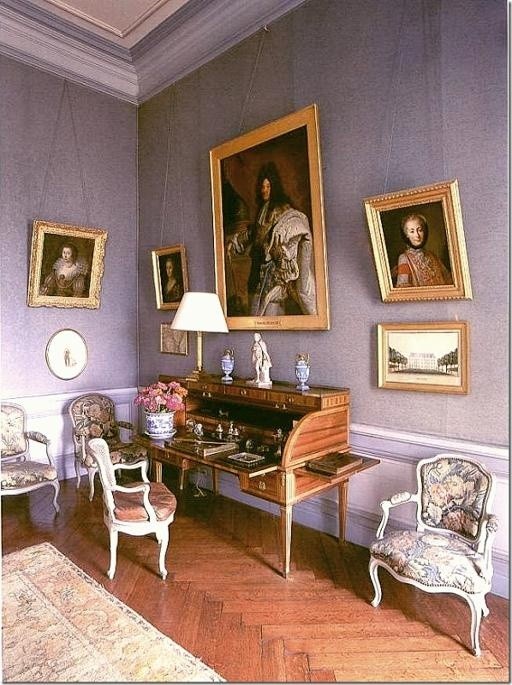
[133,381,188,412]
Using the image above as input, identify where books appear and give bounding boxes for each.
[305,451,363,474]
[294,453,380,483]
[171,432,236,457]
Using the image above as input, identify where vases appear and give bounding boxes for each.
[145,410,175,434]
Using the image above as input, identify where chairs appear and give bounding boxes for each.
[68,393,151,502]
[368,453,499,659]
[88,437,178,582]
[1,402,61,514]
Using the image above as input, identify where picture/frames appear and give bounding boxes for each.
[25,221,109,310]
[46,328,89,381]
[208,104,330,333]
[160,323,189,357]
[377,321,470,396]
[362,179,473,303]
[151,243,189,311]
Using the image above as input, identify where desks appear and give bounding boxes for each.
[129,373,380,578]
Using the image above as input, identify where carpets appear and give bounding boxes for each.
[2,541,227,685]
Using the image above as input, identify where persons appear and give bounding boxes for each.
[161,258,183,302]
[40,243,89,297]
[250,334,273,383]
[396,214,452,288]
[225,165,317,316]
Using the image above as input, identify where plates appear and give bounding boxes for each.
[144,428,176,439]
[227,452,266,464]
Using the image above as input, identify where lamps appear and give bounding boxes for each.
[169,291,230,381]
